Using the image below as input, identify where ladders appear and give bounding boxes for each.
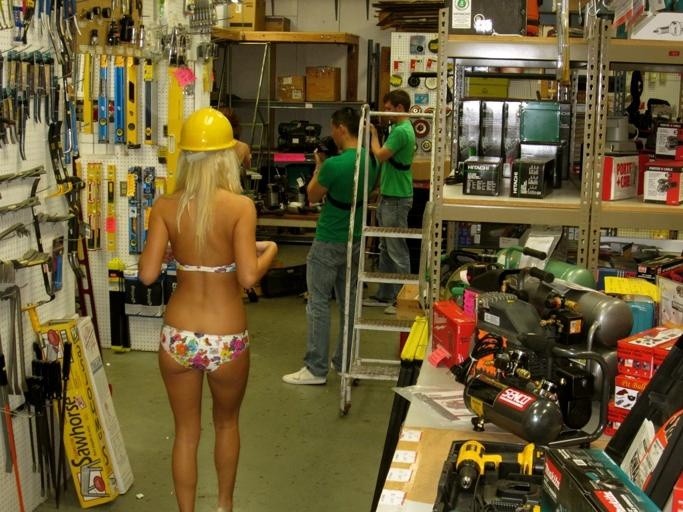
[339,103,437,417]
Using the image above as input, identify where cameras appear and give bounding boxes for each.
[364,118,387,138]
[315,136,338,157]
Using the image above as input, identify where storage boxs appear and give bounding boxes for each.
[397,281,437,324]
[223,0,291,34]
[275,66,343,105]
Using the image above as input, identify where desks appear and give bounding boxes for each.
[373,295,599,508]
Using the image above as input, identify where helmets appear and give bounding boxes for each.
[176,106,239,152]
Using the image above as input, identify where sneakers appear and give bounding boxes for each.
[361,295,394,307]
[383,300,399,314]
[329,359,342,377]
[280,364,328,386]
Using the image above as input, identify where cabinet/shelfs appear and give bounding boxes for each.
[206,26,360,246]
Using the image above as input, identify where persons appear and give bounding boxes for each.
[131,104,279,510]
[277,107,378,385]
[215,108,257,302]
[362,89,418,316]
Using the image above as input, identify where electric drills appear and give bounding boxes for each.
[455,438,536,492]
